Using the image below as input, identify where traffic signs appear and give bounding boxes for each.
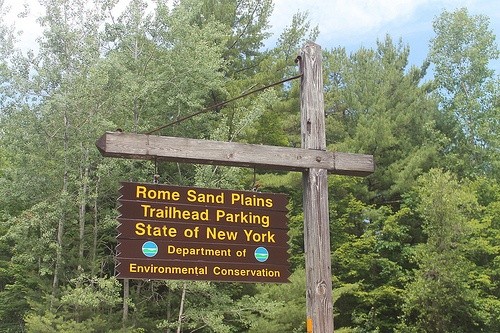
[114,180,292,284]
